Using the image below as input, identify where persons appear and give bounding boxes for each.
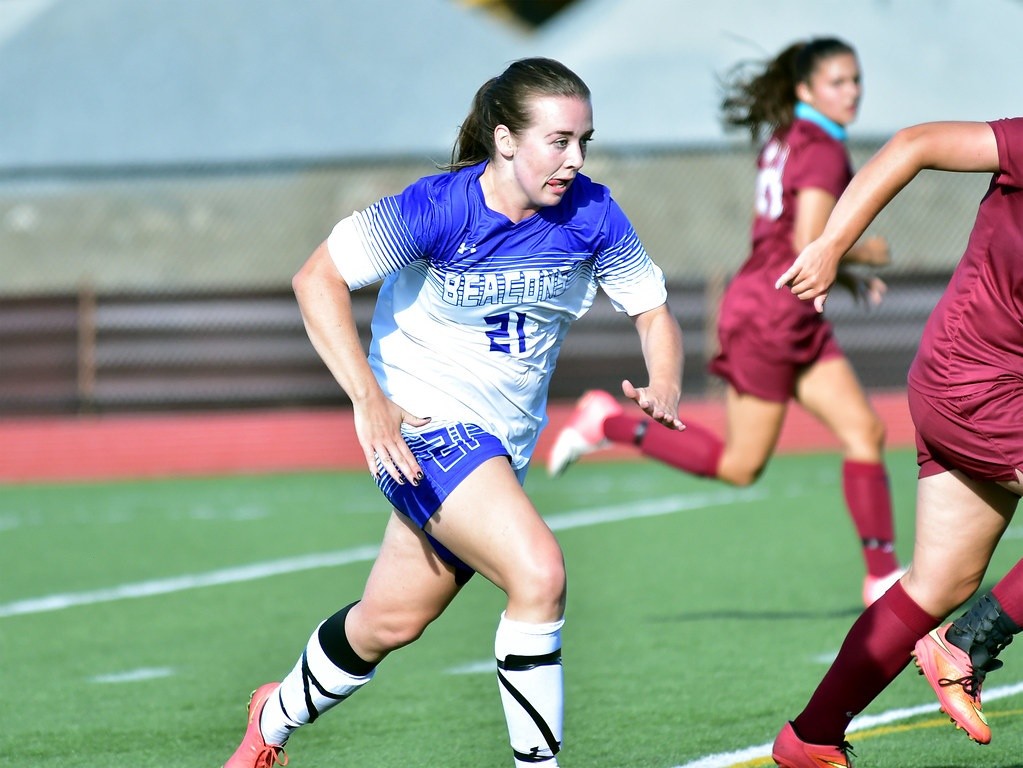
[548,37,905,607]
[223,58,684,768]
[771,118,1023,768]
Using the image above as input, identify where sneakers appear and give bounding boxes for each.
[910,621,1001,744]
[551,389,624,478]
[225,682,290,768]
[773,721,857,768]
[862,565,908,607]
